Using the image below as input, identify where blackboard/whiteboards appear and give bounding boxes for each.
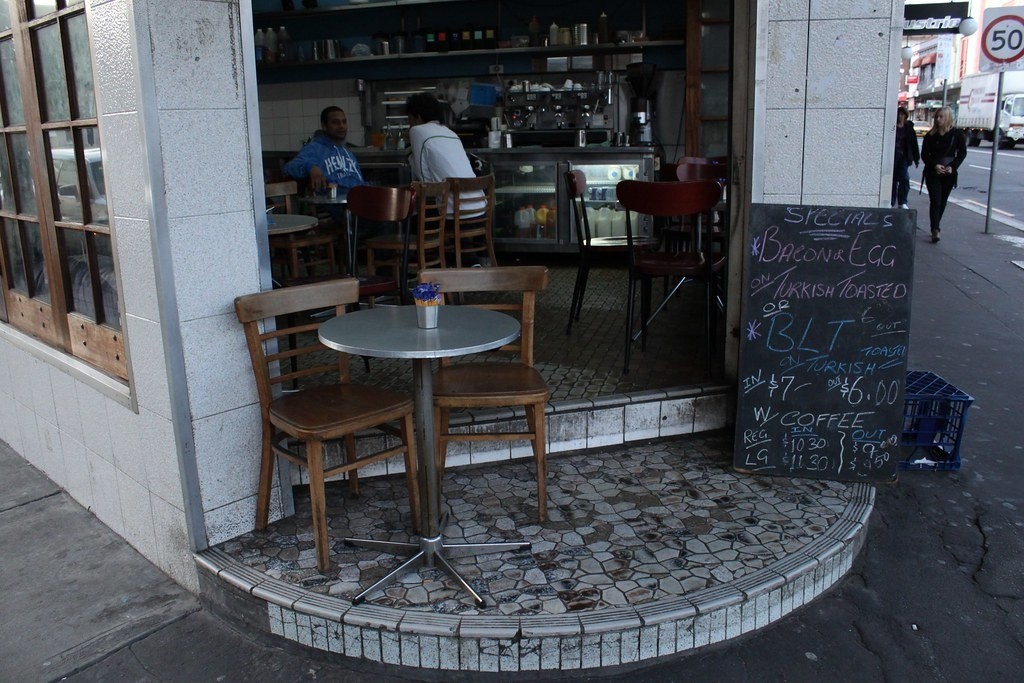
[734,203,919,484]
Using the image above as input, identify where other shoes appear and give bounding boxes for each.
[932,227,940,244]
[898,203,909,210]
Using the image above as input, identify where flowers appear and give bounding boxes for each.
[410,281,441,306]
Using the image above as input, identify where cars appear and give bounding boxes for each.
[913,121,932,137]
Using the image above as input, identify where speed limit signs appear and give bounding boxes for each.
[979,5,1024,74]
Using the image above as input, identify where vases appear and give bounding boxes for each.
[416,304,438,329]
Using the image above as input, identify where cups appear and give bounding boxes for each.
[415,305,438,329]
[575,128,586,148]
[572,18,592,45]
[327,187,337,199]
[312,39,341,61]
[615,131,630,147]
[490,117,501,131]
[617,30,645,44]
[488,131,501,148]
[371,26,498,56]
[523,81,530,92]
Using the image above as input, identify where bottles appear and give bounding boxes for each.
[513,204,556,240]
[529,16,540,47]
[549,20,559,47]
[384,121,394,152]
[396,121,405,150]
[558,28,571,46]
[254,26,290,66]
[494,96,505,123]
[598,11,610,43]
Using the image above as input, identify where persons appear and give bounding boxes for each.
[891,106,920,210]
[921,106,967,243]
[403,90,488,234]
[280,106,395,262]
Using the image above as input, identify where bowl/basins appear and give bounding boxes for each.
[510,35,529,48]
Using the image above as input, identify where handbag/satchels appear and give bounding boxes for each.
[940,156,954,167]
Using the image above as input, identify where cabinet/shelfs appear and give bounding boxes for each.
[251,0,685,72]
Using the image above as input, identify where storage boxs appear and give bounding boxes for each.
[899,369,975,472]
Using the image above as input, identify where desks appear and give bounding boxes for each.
[298,192,350,273]
[632,198,727,342]
[317,303,533,608]
[266,213,319,236]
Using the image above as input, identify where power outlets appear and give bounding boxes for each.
[503,78,517,87]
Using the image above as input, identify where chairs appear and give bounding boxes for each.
[666,155,726,327]
[265,181,336,281]
[616,179,725,379]
[304,186,415,311]
[363,178,454,300]
[562,169,660,337]
[415,173,498,304]
[417,266,550,526]
[235,277,420,574]
[275,209,372,392]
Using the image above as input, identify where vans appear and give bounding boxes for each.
[24,148,109,255]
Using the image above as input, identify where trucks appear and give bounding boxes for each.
[957,69,1024,150]
